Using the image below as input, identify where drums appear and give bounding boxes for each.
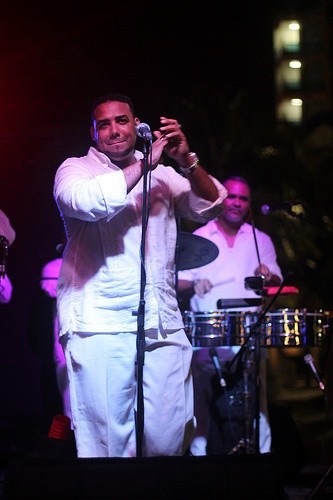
[242,310,333,348]
[182,310,263,346]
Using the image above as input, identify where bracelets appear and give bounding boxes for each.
[191,279,200,294]
[179,152,199,175]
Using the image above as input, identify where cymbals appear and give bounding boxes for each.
[174,232,220,272]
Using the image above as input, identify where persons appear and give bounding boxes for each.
[175,177,284,455]
[54,93,228,458]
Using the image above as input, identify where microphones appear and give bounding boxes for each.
[135,123,152,139]
[262,200,302,215]
[209,348,227,389]
[304,354,325,391]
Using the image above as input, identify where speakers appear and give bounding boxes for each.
[0,453,289,500]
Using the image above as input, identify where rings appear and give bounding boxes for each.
[178,125,182,129]
[160,137,163,141]
